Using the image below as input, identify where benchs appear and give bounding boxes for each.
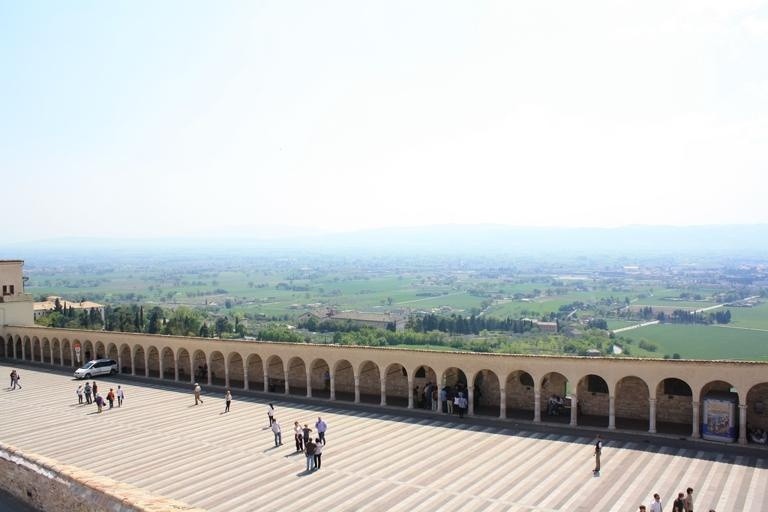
[260,376,286,391]
[193,369,216,380]
[164,366,185,376]
[548,397,583,418]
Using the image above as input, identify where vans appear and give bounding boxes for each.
[73,358,119,378]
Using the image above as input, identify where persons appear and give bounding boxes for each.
[10,370,14,386]
[267,403,274,427]
[548,394,564,415]
[12,370,22,390]
[272,419,283,447]
[224,391,232,412]
[592,434,602,472]
[413,382,467,418]
[293,416,327,472]
[77,381,124,413]
[639,487,694,512]
[194,383,203,405]
